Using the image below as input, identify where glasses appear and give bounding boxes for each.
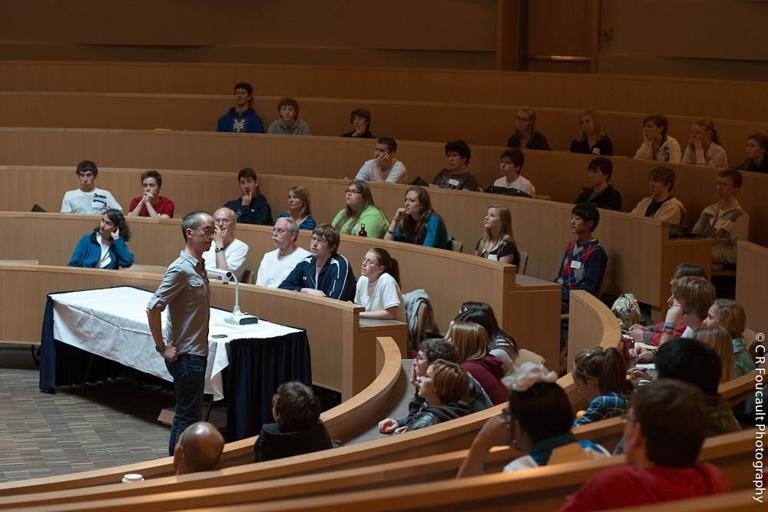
[206,229,217,237]
[361,257,381,267]
[416,355,428,362]
[502,407,516,422]
[345,189,361,194]
[272,227,285,234]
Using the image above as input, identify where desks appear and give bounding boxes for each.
[516,275,562,376]
[337,358,440,446]
[669,236,712,303]
[359,316,408,398]
[39,284,313,442]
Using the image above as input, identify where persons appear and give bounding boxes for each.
[626,326,733,384]
[702,298,757,379]
[67,208,135,270]
[253,381,333,462]
[454,308,518,373]
[658,276,716,348]
[146,210,216,456]
[446,302,521,357]
[557,380,730,512]
[217,81,264,133]
[633,114,682,163]
[127,170,176,219]
[574,156,622,211]
[695,169,750,270]
[614,337,742,455]
[394,358,471,436]
[340,108,375,138]
[553,203,608,326]
[256,217,312,289]
[455,381,610,480]
[383,187,448,250]
[569,110,613,155]
[442,324,512,405]
[572,346,635,427]
[354,136,407,185]
[272,185,316,230]
[738,133,768,173]
[202,207,250,282]
[629,166,688,225]
[277,223,356,301]
[506,106,551,151]
[60,160,122,214]
[173,422,224,475]
[681,118,727,167]
[628,263,708,344]
[347,248,403,321]
[330,180,390,238]
[427,138,477,191]
[493,149,535,198]
[267,98,310,136]
[474,204,520,273]
[377,338,494,435]
[221,168,272,225]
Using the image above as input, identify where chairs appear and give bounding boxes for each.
[517,249,529,275]
[562,259,616,321]
[242,268,254,283]
[450,238,463,252]
[399,293,428,355]
[742,327,760,358]
